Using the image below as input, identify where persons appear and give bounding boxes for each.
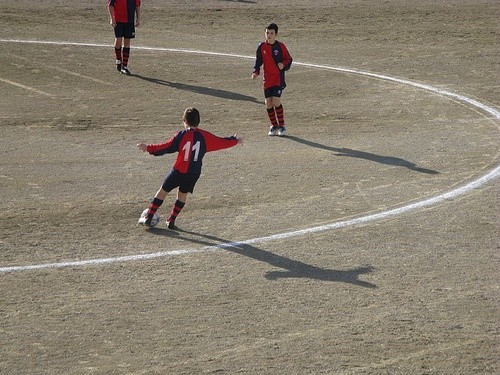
[251,21,294,138]
[105,0,142,75]
[137,108,244,230]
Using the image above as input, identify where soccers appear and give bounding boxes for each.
[140,208,160,227]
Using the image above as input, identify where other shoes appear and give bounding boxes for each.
[138,218,151,225]
[278,126,285,136]
[268,126,278,135]
[121,65,130,75]
[116,58,122,71]
[166,220,174,229]
